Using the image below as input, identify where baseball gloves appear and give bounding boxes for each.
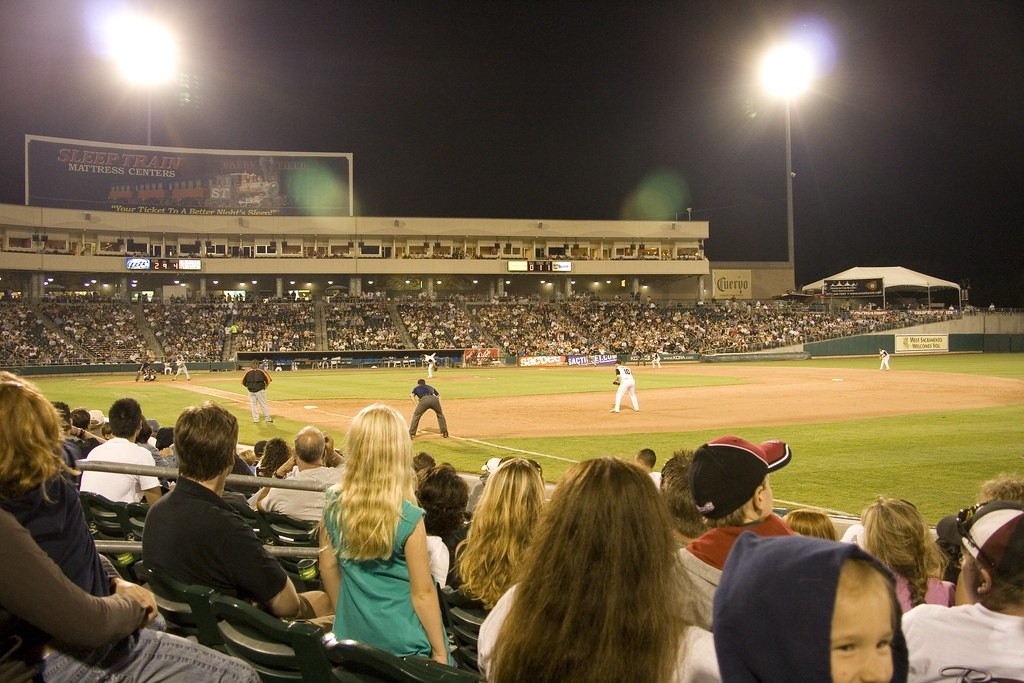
[612,380,620,386]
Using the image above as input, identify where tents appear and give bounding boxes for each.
[803,265,961,312]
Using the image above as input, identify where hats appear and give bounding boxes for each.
[615,359,621,364]
[961,500,1024,578]
[88,410,105,427]
[689,433,792,519]
[481,458,501,474]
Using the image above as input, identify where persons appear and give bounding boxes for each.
[878,348,890,370]
[312,361,319,369]
[135,357,150,381]
[275,362,283,372]
[0,370,1024,683]
[322,355,329,370]
[164,361,172,375]
[172,354,189,380]
[610,359,639,411]
[650,351,661,368]
[291,359,298,370]
[241,359,273,423]
[263,358,268,371]
[0,288,995,367]
[144,368,157,380]
[423,352,435,378]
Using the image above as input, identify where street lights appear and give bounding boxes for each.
[109,22,177,147]
[758,42,815,261]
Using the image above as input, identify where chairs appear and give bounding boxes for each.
[79,491,493,683]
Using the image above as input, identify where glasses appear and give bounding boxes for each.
[499,456,544,487]
[485,461,490,471]
[957,502,994,566]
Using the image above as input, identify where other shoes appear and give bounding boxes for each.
[610,410,619,413]
[443,432,448,438]
